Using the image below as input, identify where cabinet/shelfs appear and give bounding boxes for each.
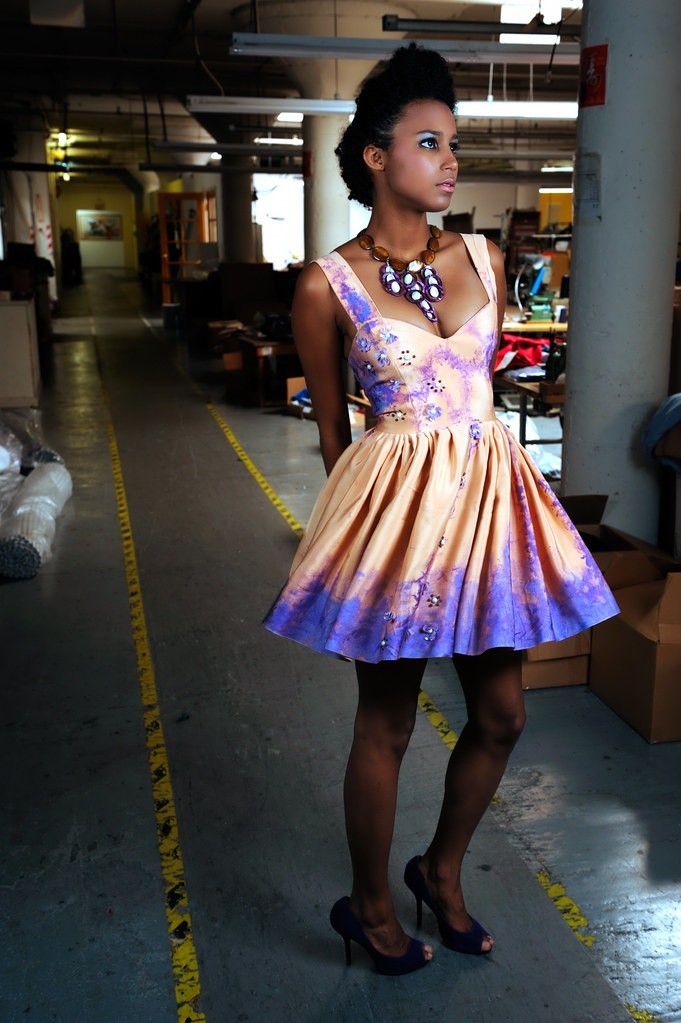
[0,297,43,408]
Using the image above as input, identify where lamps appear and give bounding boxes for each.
[136,0,582,186]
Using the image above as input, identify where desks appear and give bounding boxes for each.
[237,333,304,409]
[501,320,567,335]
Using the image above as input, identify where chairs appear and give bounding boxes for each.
[509,328,567,450]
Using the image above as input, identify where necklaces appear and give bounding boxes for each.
[357,223,445,322]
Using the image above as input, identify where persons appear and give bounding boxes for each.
[292,44,621,974]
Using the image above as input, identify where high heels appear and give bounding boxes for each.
[331,896,434,976]
[402,854,494,956]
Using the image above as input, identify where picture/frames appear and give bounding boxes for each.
[76,209,124,241]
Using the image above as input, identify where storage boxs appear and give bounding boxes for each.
[523,492,681,662]
[522,656,589,690]
[589,573,681,745]
